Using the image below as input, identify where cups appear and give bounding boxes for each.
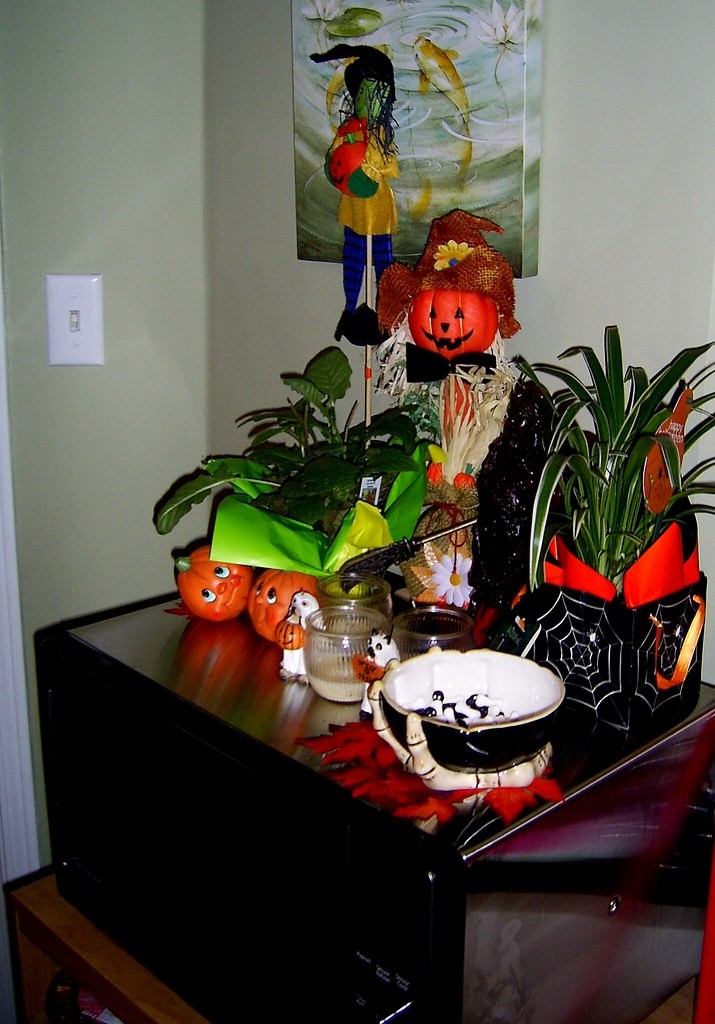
[304,571,477,706]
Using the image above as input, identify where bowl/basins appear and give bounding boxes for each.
[380,645,566,769]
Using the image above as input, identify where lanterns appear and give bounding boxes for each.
[175,545,322,642]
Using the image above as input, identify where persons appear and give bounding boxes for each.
[376,208,522,605]
[309,44,401,345]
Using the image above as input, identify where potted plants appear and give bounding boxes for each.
[510,326,715,752]
[157,347,432,597]
